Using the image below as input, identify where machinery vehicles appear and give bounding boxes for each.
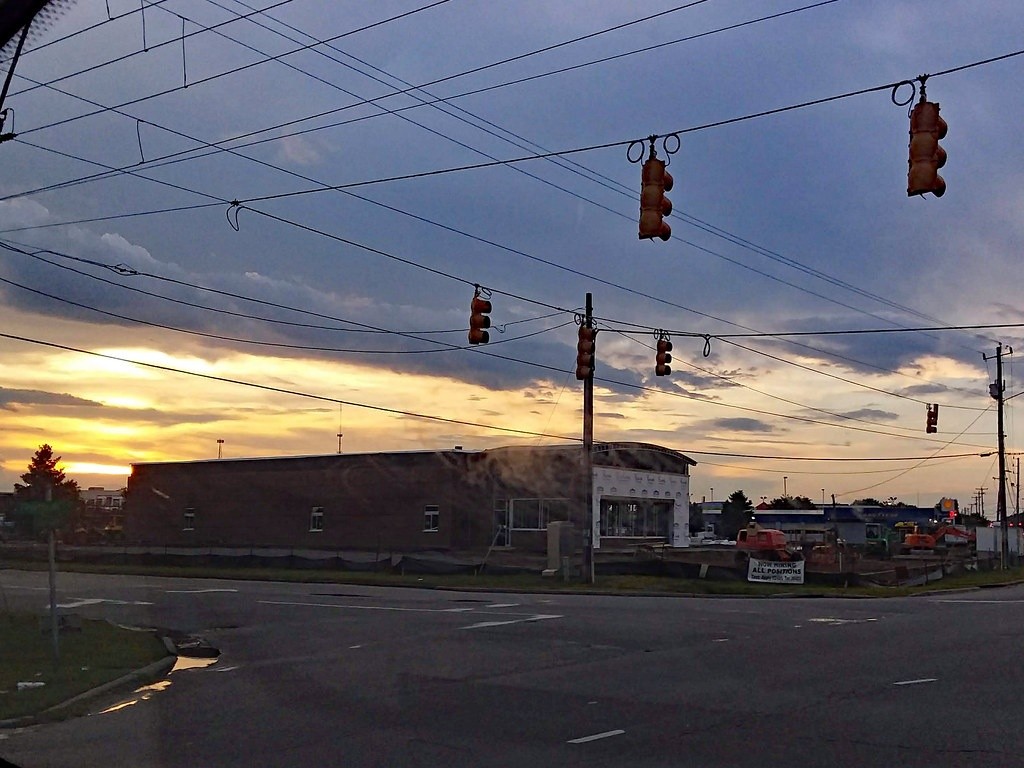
[864,523,891,560]
[733,528,806,582]
[897,527,977,555]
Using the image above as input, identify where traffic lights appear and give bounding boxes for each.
[927,410,939,434]
[655,339,675,378]
[639,158,678,246]
[907,101,949,200]
[575,327,597,380]
[468,297,491,347]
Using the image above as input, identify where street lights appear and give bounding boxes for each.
[710,488,713,502]
[821,489,825,504]
[783,477,788,496]
[760,496,768,503]
